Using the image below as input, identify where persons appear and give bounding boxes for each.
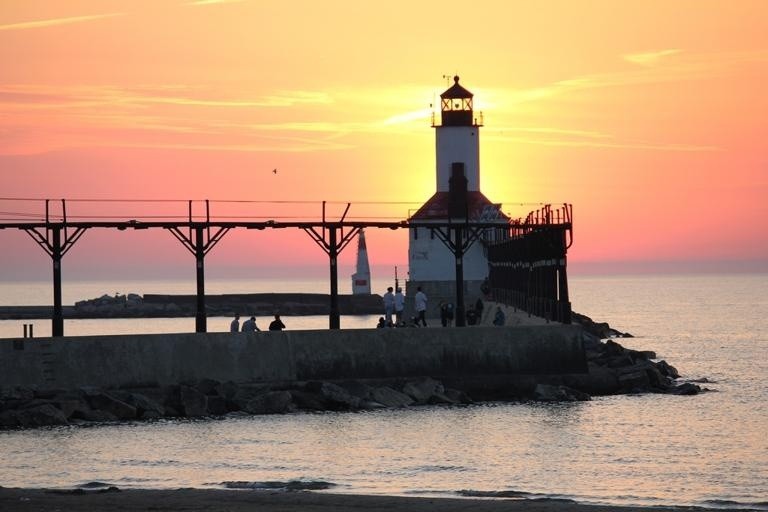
[241,316,261,332]
[230,314,240,332]
[268,314,285,331]
[377,285,504,329]
